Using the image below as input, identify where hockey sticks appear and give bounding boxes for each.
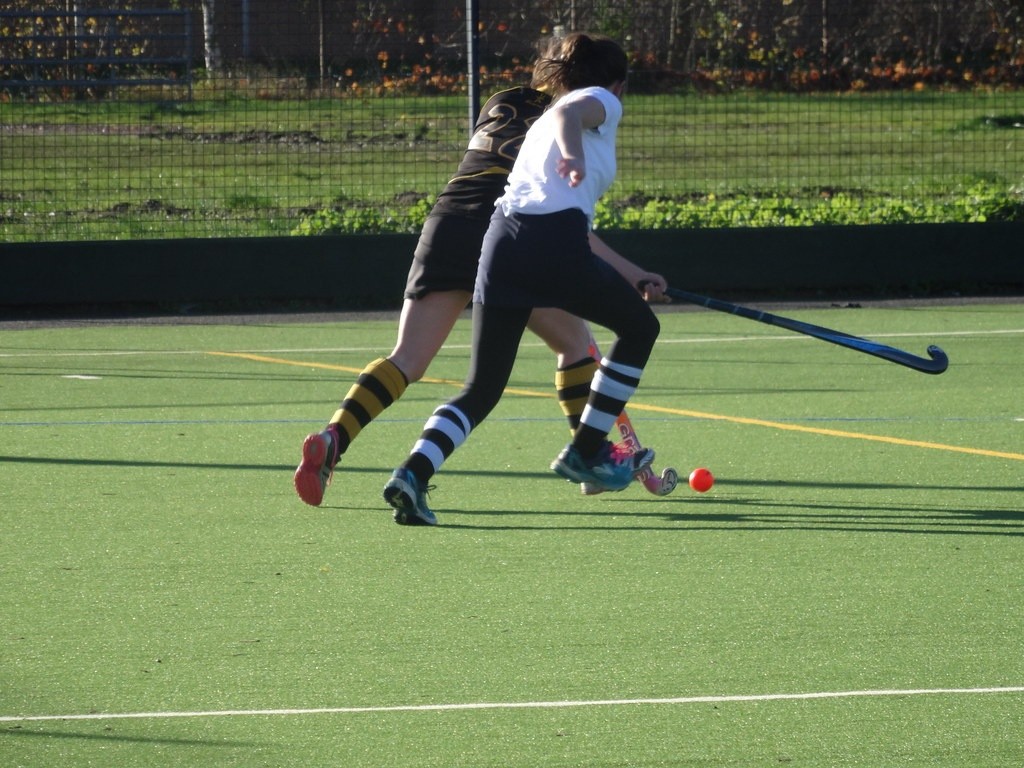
[634,277,952,376]
[581,318,680,497]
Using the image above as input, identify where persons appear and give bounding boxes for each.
[293,31,671,527]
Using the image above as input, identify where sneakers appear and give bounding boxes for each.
[580,447,655,495]
[381,467,438,525]
[549,441,635,491]
[293,424,342,506]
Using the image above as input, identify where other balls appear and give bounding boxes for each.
[687,467,716,493]
